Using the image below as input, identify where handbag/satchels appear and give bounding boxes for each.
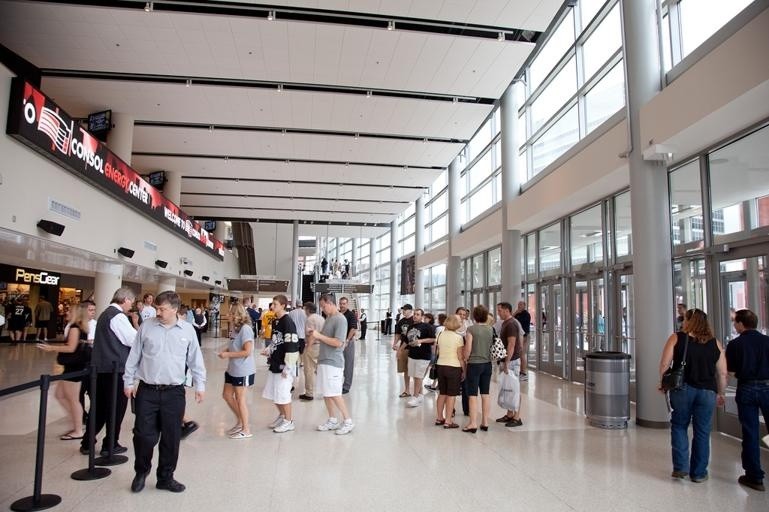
[490,338,507,362]
[430,365,437,379]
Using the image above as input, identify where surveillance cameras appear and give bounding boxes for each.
[180,255,189,267]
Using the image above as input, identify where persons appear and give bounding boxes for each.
[177,304,220,347]
[123,290,206,492]
[358,308,367,340]
[726,310,769,491]
[125,293,199,446]
[541,307,627,351]
[313,258,350,279]
[217,294,358,439]
[80,287,138,456]
[384,308,394,336]
[392,301,531,433]
[658,309,728,482]
[1,296,72,346]
[676,304,686,330]
[35,299,99,440]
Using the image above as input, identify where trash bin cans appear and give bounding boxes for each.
[582,351,632,430]
[381,320,386,333]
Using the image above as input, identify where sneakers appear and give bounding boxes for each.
[399,385,436,406]
[231,432,251,438]
[335,423,354,434]
[80,446,90,454]
[131,470,149,491]
[506,419,522,427]
[270,416,285,427]
[316,421,339,430]
[496,415,513,422]
[156,479,185,492]
[227,424,243,434]
[299,395,313,400]
[100,446,126,455]
[274,419,295,432]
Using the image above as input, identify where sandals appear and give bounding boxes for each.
[435,408,488,433]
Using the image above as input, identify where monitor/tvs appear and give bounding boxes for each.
[205,221,216,232]
[86,109,112,132]
[147,170,166,187]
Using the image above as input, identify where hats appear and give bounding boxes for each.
[401,304,412,309]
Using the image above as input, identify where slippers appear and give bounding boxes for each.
[62,433,84,440]
